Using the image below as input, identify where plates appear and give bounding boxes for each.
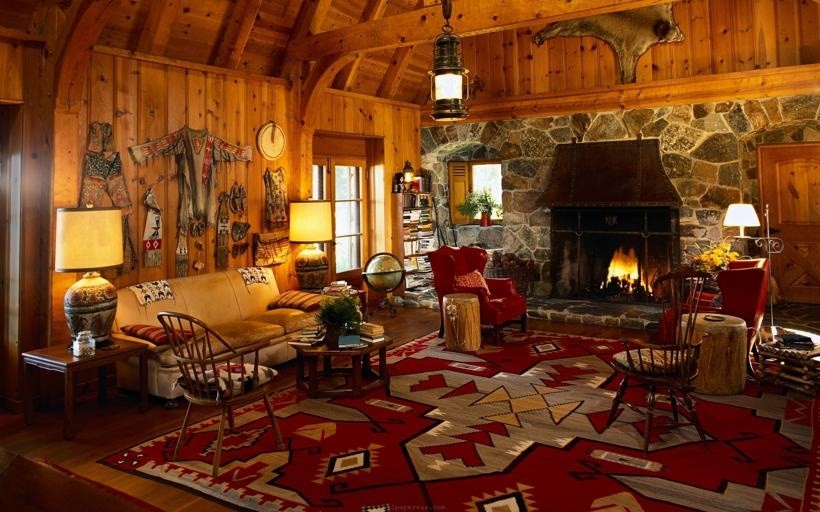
[704,314,724,321]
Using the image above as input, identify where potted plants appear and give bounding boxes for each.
[454,185,506,228]
[313,285,363,349]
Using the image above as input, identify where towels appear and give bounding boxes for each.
[125,279,175,308]
[234,266,270,287]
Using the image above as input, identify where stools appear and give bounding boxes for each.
[440,292,482,354]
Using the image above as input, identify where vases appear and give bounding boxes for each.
[701,267,726,307]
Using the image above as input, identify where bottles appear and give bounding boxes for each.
[74,331,96,357]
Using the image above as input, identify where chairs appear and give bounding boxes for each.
[154,309,290,480]
[425,243,529,348]
[604,267,714,462]
[656,255,773,378]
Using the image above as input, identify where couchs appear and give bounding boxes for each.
[103,265,365,413]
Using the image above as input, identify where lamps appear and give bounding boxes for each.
[286,199,336,296]
[53,203,128,353]
[723,199,786,328]
[425,0,472,124]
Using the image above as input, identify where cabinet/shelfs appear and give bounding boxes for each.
[389,191,438,308]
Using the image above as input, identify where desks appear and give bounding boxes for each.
[320,285,369,324]
[673,309,748,397]
[756,326,820,400]
[18,333,151,441]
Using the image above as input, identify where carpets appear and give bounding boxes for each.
[89,319,820,512]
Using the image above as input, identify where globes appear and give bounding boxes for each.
[361,252,406,317]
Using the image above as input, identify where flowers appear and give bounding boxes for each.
[685,234,747,275]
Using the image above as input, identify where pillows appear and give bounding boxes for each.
[265,288,325,312]
[708,289,725,308]
[118,321,198,348]
[451,268,493,298]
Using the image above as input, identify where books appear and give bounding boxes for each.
[323,281,353,296]
[288,321,385,348]
[773,334,815,351]
[404,194,440,311]
